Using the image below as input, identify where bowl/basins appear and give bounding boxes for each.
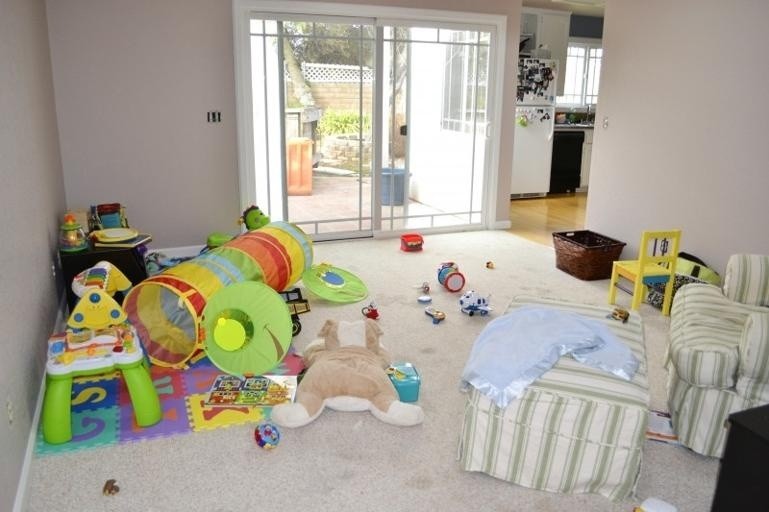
[531,48,552,58]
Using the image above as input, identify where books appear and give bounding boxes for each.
[94,235,151,248]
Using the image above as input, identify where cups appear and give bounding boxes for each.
[568,113,576,124]
[556,114,566,124]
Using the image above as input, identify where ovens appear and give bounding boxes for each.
[553,131,585,195]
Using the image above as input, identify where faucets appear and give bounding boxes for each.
[585,105,591,123]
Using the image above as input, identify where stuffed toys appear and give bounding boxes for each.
[270,318,424,430]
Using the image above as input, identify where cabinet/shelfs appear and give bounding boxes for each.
[518,13,537,56]
[547,131,585,194]
[535,14,570,96]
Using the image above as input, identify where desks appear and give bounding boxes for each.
[708,404,768,512]
[59,231,149,317]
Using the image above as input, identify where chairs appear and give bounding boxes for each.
[608,229,682,317]
[660,251,768,458]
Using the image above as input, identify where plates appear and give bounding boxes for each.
[89,226,137,242]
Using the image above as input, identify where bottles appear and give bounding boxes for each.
[361,301,381,319]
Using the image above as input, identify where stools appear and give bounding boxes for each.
[456,292,651,504]
[41,324,162,445]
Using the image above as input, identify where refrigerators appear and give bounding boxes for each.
[511,55,560,200]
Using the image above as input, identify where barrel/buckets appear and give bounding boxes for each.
[370,168,411,206]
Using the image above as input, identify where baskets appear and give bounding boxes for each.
[552,229,627,281]
